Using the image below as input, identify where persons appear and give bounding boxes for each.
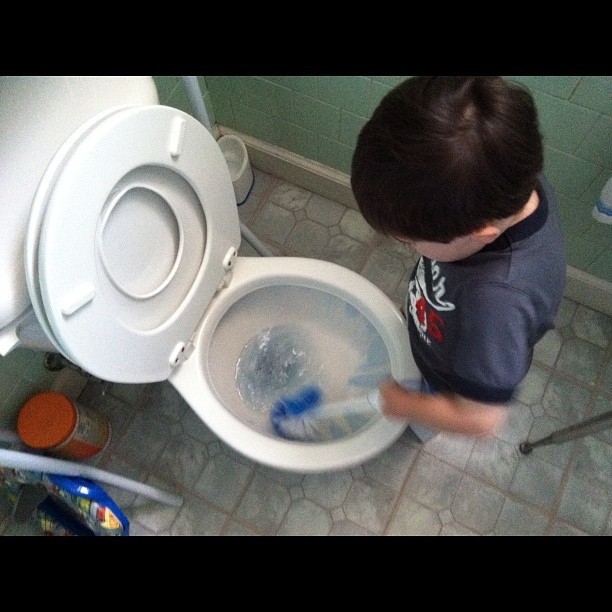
[351,74,571,444]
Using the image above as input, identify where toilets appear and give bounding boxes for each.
[1,75,423,474]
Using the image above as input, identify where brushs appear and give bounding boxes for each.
[270,380,417,442]
